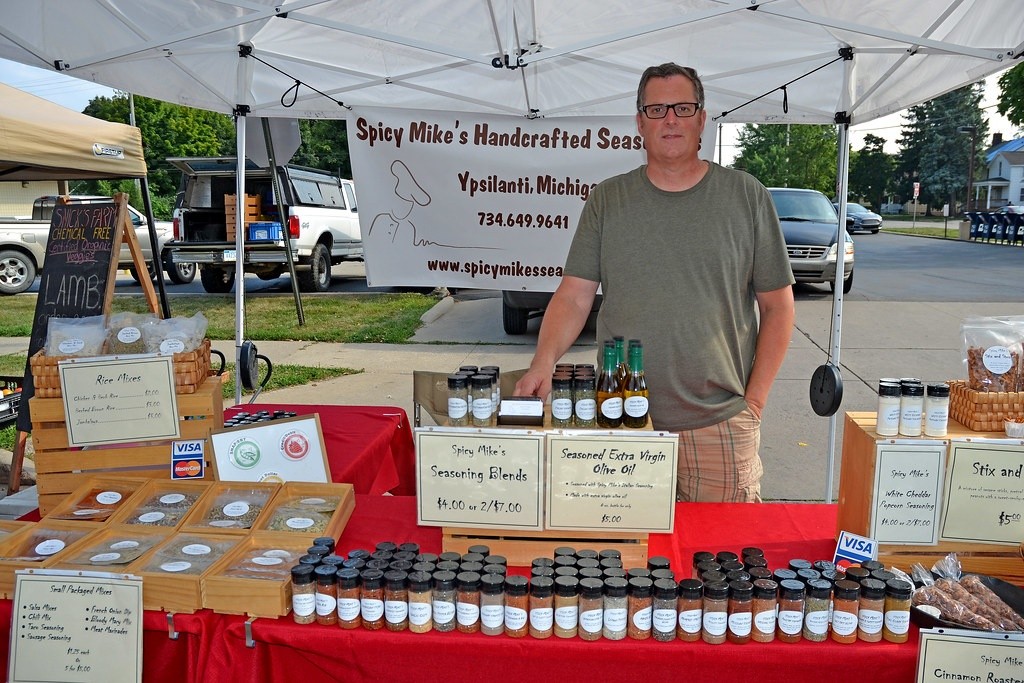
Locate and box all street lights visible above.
[956,125,977,213]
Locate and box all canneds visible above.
[875,378,951,438]
[551,363,597,428]
[447,365,501,427]
[291,536,913,644]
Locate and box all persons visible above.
[512,62,794,503]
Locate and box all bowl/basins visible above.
[908,569,1024,634]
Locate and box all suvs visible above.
[765,187,856,294]
[160,155,365,294]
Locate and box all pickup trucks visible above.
[0,194,197,297]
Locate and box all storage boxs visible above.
[833,410,1024,587]
[224,193,261,243]
[28,376,226,519]
[249,222,284,241]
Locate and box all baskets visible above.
[946,380,1024,432]
[31,336,211,397]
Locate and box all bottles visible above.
[291,537,913,644]
[875,377,925,437]
[595,335,649,429]
[223,409,296,430]
[551,362,596,428]
[447,365,501,427]
[924,383,950,438]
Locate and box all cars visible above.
[498,290,602,337]
[832,201,883,235]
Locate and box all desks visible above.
[1,403,1024,683]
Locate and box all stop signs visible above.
[914,183,920,196]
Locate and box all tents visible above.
[0,0,1024,502]
[0,81,172,321]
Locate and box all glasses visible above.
[640,102,701,119]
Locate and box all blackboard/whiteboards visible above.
[15,192,130,433]
